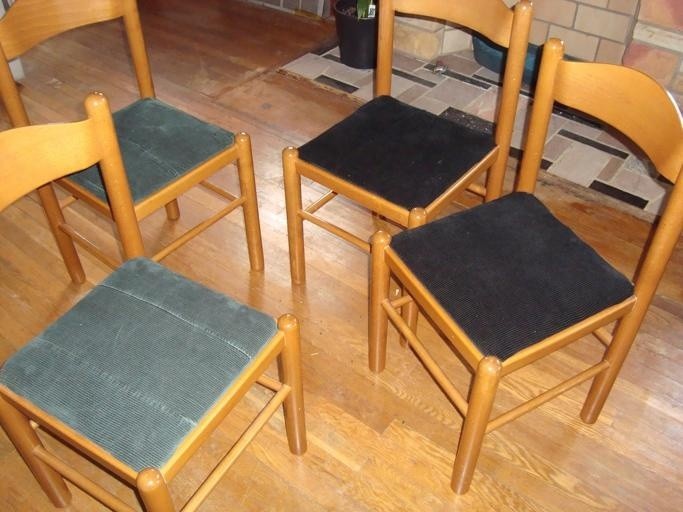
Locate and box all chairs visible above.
[281,0,534,350]
[368,37,683,492]
[0,92,307,511]
[1,0,265,285]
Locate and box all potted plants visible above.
[331,0,380,68]
